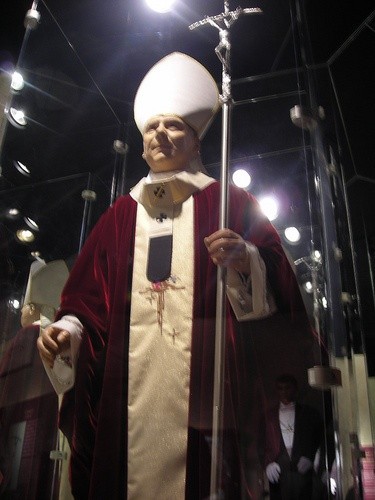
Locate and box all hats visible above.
[24,260,69,310]
[133,50,220,140]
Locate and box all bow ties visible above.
[281,401,296,409]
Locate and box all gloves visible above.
[297,456,312,474]
[266,462,280,481]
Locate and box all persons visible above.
[34,50,334,500]
[0,258,69,500]
[257,370,328,500]
